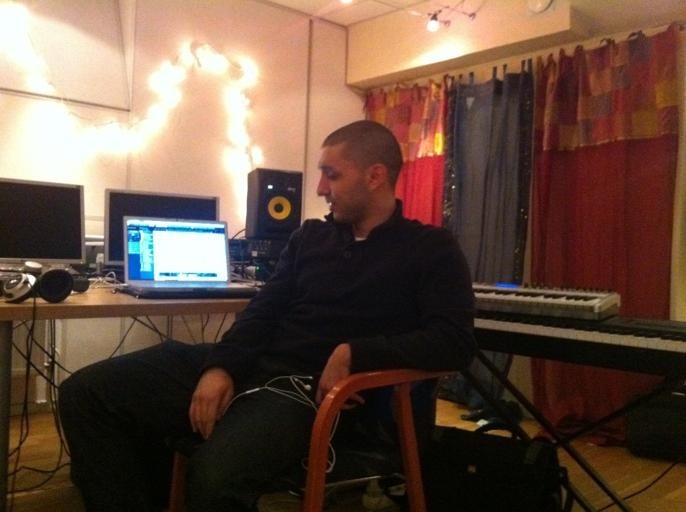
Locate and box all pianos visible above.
[472,280,621,321]
[474,317,686,374]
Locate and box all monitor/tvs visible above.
[0,178,86,265]
[104,188,220,267]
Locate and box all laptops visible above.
[122,216,259,299]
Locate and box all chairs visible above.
[169,369,454,511]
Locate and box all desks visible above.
[0,279,249,512]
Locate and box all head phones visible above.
[3,269,72,304]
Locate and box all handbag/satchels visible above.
[415,421,571,512]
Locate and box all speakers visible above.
[246,168,301,239]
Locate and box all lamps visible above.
[425,5,449,34]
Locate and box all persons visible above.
[58,120,475,510]
